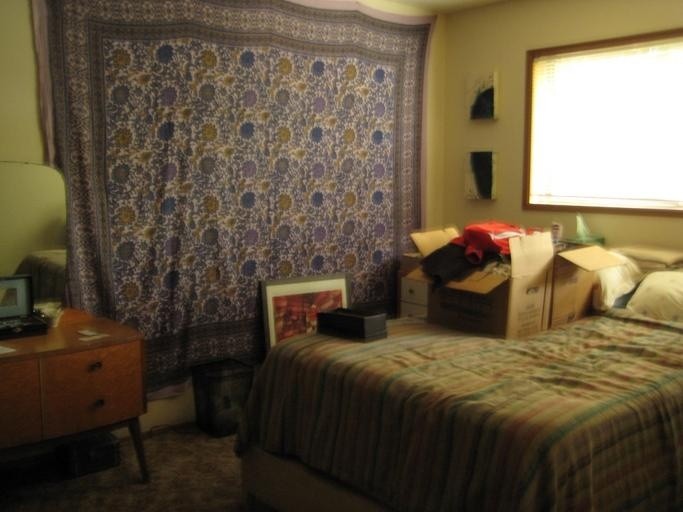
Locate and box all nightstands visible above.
[397,271,433,319]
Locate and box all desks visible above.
[0,308,150,483]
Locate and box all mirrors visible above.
[0,160,68,309]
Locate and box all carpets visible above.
[0,421,242,512]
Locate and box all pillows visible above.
[616,271,683,330]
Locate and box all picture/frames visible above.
[0,274,33,321]
[260,272,352,351]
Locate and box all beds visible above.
[234,247,683,512]
[13,249,67,303]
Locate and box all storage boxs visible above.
[410,227,629,338]
